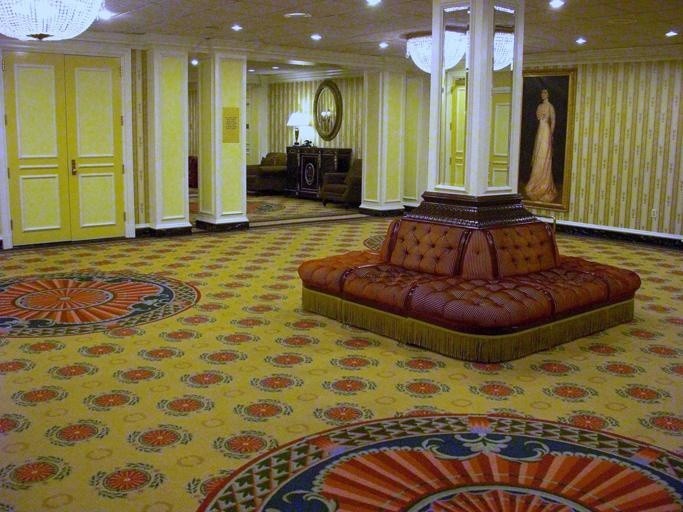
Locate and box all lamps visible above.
[0,0,102,41]
[402,27,466,75]
[286,112,308,147]
[466,26,514,72]
[320,110,330,122]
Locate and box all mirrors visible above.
[312,79,343,142]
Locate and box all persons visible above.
[523,85,561,204]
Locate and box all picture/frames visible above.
[522,65,578,213]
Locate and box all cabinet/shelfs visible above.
[283,147,351,201]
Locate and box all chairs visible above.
[246,152,288,197]
[318,159,362,210]
[188,156,198,188]
[298,216,642,363]
